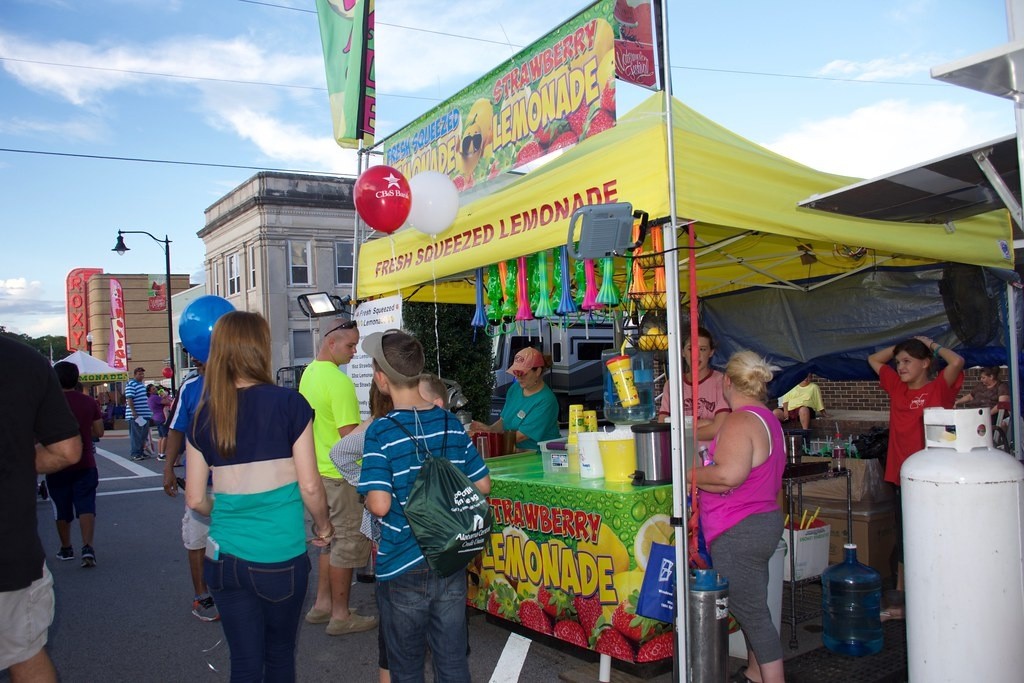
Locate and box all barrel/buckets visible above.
[628,420,673,485]
[599,440,637,482]
[578,432,608,479]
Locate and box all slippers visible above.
[729,665,760,683]
[880,607,905,622]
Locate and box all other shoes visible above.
[156,455,167,461]
[56,545,75,560]
[129,452,150,460]
[80,544,96,567]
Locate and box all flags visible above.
[316,0,375,149]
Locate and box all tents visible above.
[52,350,129,407]
[350,90,1024,683]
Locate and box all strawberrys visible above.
[727,613,740,633]
[454,77,616,191]
[468,582,674,662]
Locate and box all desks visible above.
[468,449,678,682]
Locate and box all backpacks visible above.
[386,412,494,579]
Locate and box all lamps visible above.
[297,292,351,318]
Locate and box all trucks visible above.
[488,314,649,429]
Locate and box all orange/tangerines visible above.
[502,514,676,622]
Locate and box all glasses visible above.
[325,319,357,336]
[147,384,152,391]
[137,368,145,372]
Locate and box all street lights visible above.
[86,332,95,398]
[43,338,53,366]
[109,229,176,400]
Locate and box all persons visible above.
[35,361,105,569]
[867,335,964,622]
[772,372,834,430]
[186,312,335,683]
[0,334,82,683]
[657,327,732,466]
[952,366,1010,442]
[330,329,491,683]
[125,347,220,622]
[467,347,562,453]
[297,319,380,636]
[685,352,786,683]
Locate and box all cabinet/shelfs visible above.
[782,469,853,654]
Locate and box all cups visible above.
[607,355,639,408]
[569,405,598,473]
[473,429,518,458]
[786,435,802,467]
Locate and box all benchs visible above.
[811,410,889,428]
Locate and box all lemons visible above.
[638,326,669,351]
[435,18,615,172]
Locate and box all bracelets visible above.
[930,342,936,353]
[305,521,335,545]
[933,343,942,359]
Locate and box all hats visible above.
[505,347,545,378]
[362,328,426,383]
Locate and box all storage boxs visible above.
[536,437,569,473]
[786,456,883,501]
[784,497,902,566]
[782,524,830,582]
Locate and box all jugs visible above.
[664,416,698,469]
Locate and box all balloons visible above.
[406,170,459,238]
[353,165,411,235]
[179,295,237,364]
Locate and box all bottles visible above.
[832,432,846,477]
[700,446,734,496]
[821,542,884,656]
[602,349,657,426]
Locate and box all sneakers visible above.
[191,594,220,621]
[305,605,333,624]
[325,608,376,635]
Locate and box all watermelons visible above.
[613,0,656,89]
